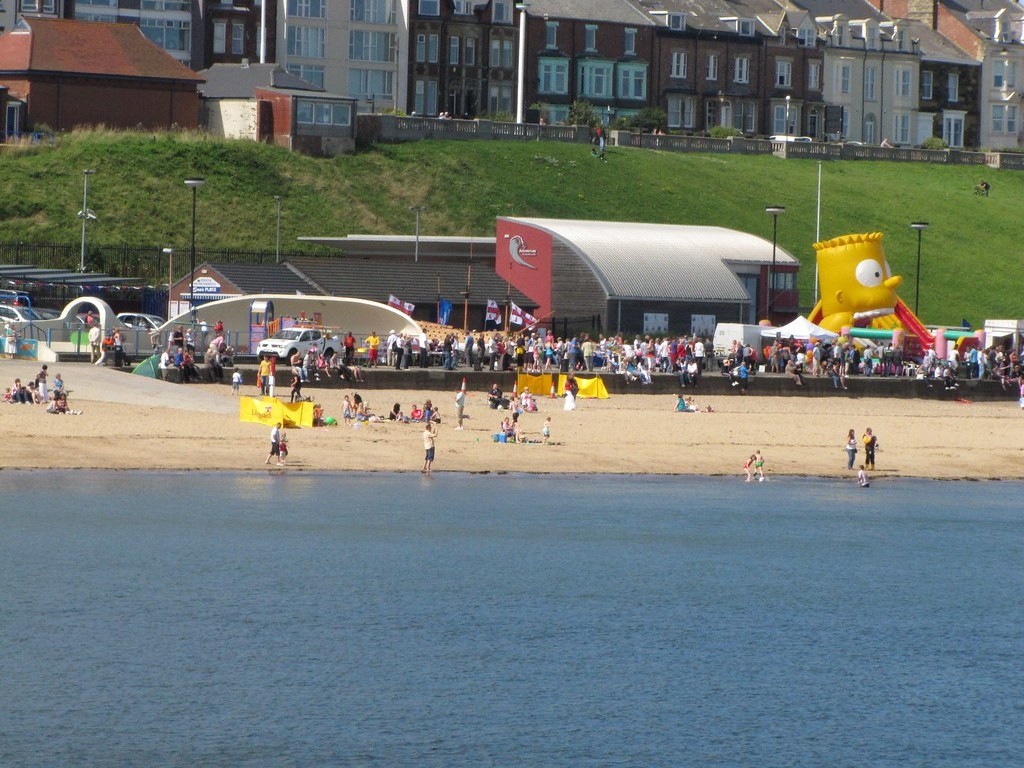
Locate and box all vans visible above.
[714,324,783,365]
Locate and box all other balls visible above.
[325,417,335,424]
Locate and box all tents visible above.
[760,316,839,344]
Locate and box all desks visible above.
[392,352,420,366]
[429,351,453,367]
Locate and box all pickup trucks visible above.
[256,329,342,368]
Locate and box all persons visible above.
[979,179,990,197]
[652,128,665,148]
[597,124,606,152]
[591,148,605,159]
[2,311,1024,486]
[437,112,452,120]
[880,138,892,147]
[421,424,439,474]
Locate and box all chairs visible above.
[352,347,368,366]
[903,361,917,377]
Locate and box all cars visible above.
[0,302,102,331]
[117,314,164,329]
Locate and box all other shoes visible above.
[277,463,284,466]
[266,461,270,465]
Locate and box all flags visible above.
[439,298,452,325]
[486,299,502,325]
[509,301,537,331]
[388,294,415,317]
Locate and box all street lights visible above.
[273,195,283,262]
[80,168,96,273]
[516,2,531,130]
[183,178,206,305]
[910,221,929,316]
[765,205,785,325]
[163,248,174,319]
[786,94,791,137]
[409,205,427,261]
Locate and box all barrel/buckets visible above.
[499,434,507,443]
[493,433,499,442]
